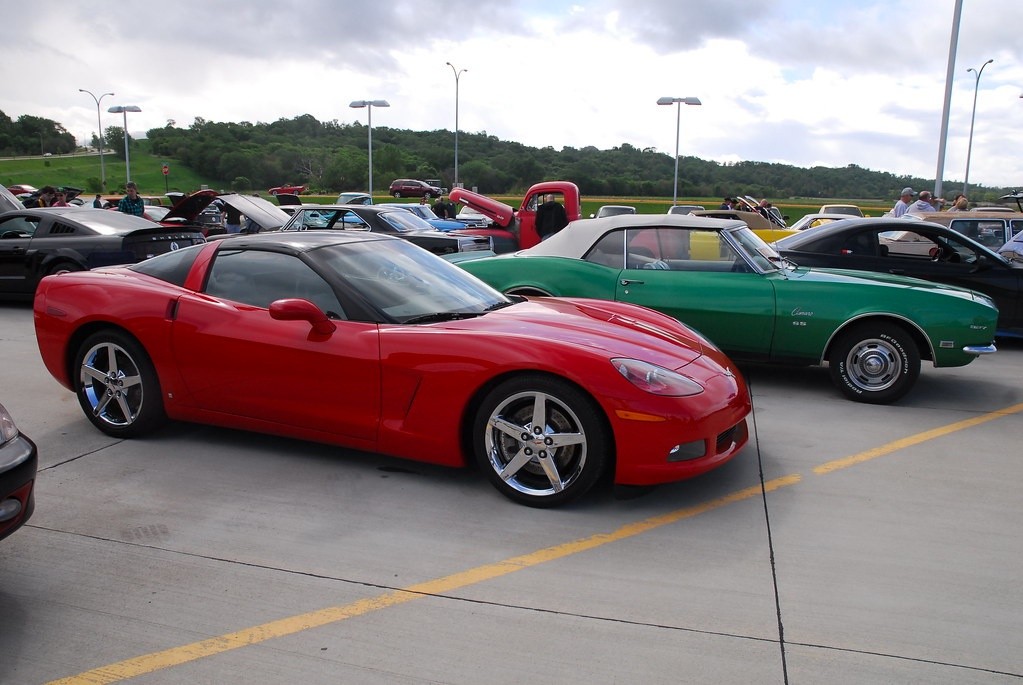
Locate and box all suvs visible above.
[388,179,443,200]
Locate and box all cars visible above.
[44,152,52,157]
[0,403,39,541]
[1,180,1023,259]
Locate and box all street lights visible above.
[80,88,116,191]
[349,100,390,196]
[657,96,702,205]
[964,58,994,195]
[108,105,142,184]
[446,61,467,186]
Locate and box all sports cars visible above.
[1,188,221,307]
[763,217,1023,352]
[433,214,1001,406]
[33,229,754,509]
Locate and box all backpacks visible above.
[882,208,895,218]
[22,198,45,209]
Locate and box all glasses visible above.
[46,194,53,197]
[911,195,914,197]
[930,197,931,199]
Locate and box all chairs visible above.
[878,244,889,257]
[1,231,21,240]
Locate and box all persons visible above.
[93,194,102,208]
[432,197,456,219]
[890,187,943,217]
[720,197,767,213]
[221,192,261,233]
[535,194,568,240]
[947,194,969,213]
[24,185,70,208]
[118,182,144,216]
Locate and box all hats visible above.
[731,199,738,203]
[56,187,68,193]
[96,194,102,198]
[901,187,918,197]
[724,196,731,201]
[127,181,136,190]
[931,193,938,199]
[435,199,441,203]
